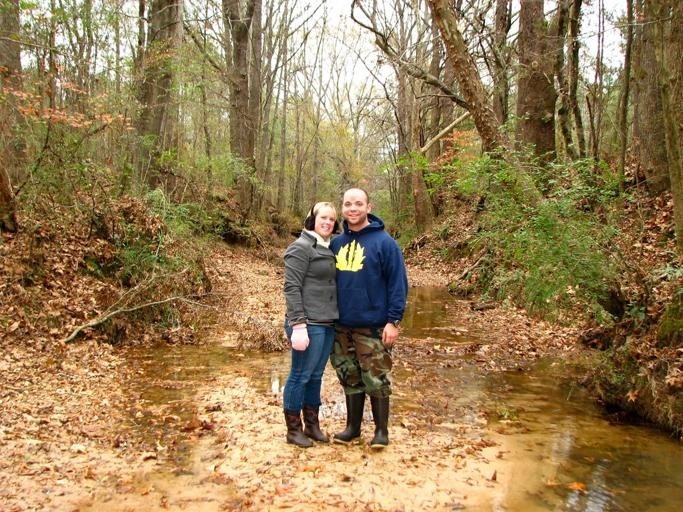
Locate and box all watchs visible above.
[388,319,400,327]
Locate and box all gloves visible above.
[291,324,310,352]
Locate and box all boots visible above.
[334,392,365,444]
[284,411,313,447]
[370,395,389,449]
[302,406,329,442]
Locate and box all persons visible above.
[281,200,343,448]
[328,186,410,450]
